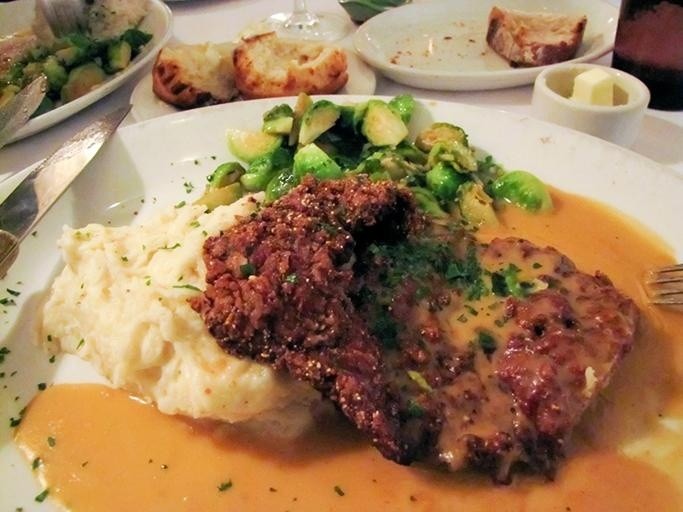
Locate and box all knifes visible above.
[0,74,49,149]
[0,104,132,280]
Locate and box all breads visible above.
[485,6,587,66]
[231,31,348,97]
[150,39,241,108]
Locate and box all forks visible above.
[645,264,683,304]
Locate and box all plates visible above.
[0,93,683,512]
[352,0,619,91]
[0,0,174,146]
[533,60,651,151]
[127,0,376,123]
